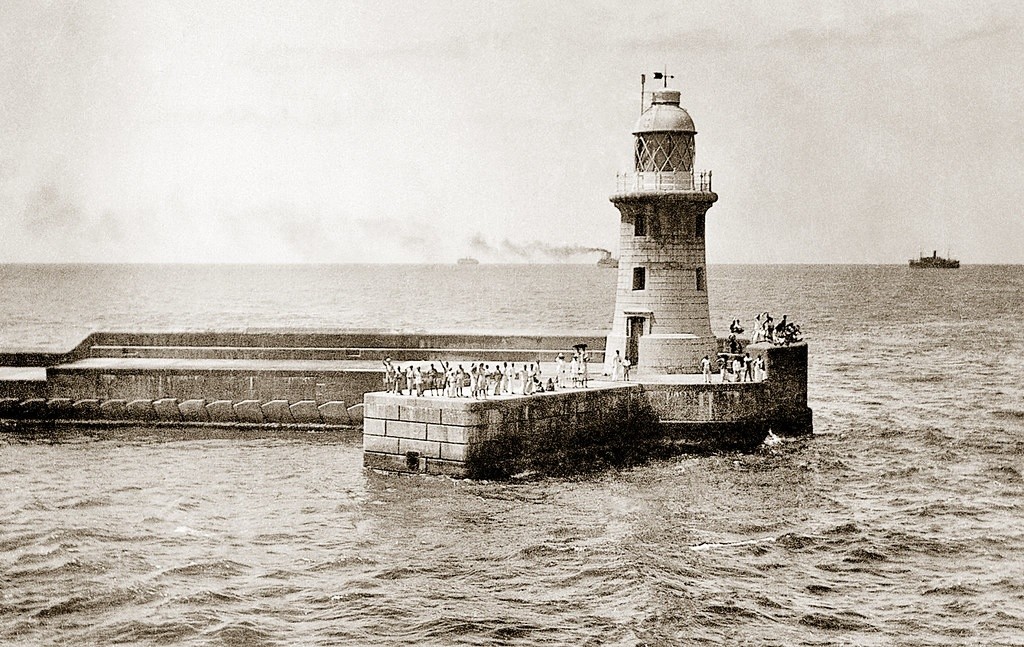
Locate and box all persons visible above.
[700,354,712,383]
[716,353,768,382]
[518,359,545,395]
[611,350,623,381]
[622,355,633,381]
[381,355,465,398]
[470,361,517,399]
[753,311,803,346]
[730,319,745,334]
[546,344,591,391]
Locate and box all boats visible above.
[458,257,479,266]
[908,250,962,270]
[596,250,619,267]
